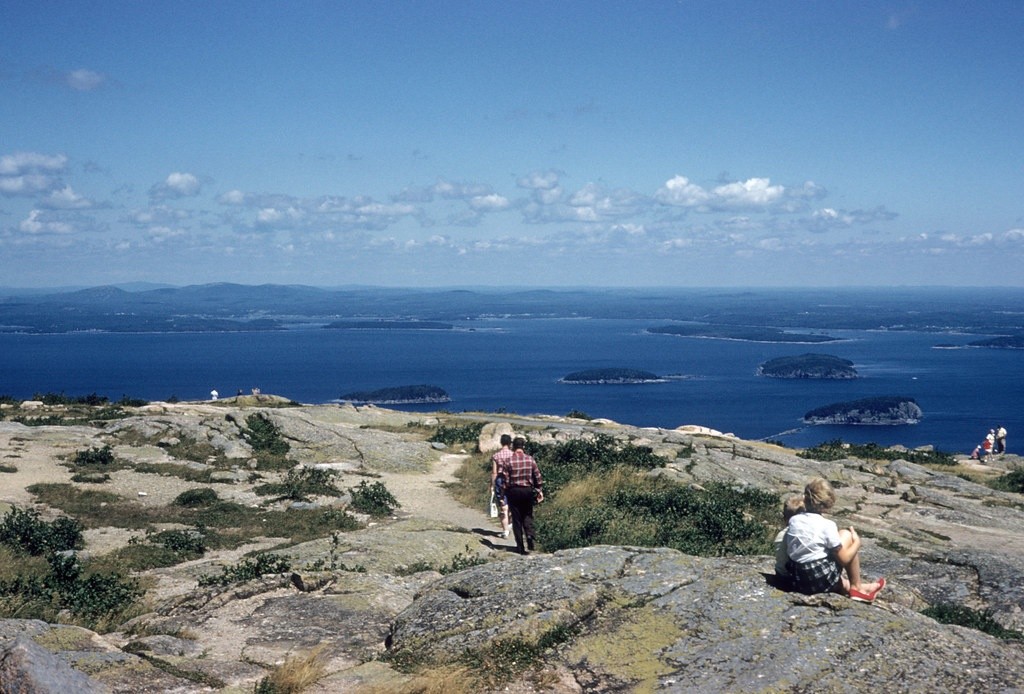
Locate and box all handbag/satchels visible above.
[490,492,498,518]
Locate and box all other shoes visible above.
[502,530,510,539]
[516,548,525,554]
[849,589,874,603]
[527,535,535,550]
[870,578,886,599]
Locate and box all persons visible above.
[499,437,544,554]
[971,424,1007,462]
[774,480,886,602]
[490,434,513,539]
[211,388,219,401]
[237,389,242,396]
[252,387,260,395]
